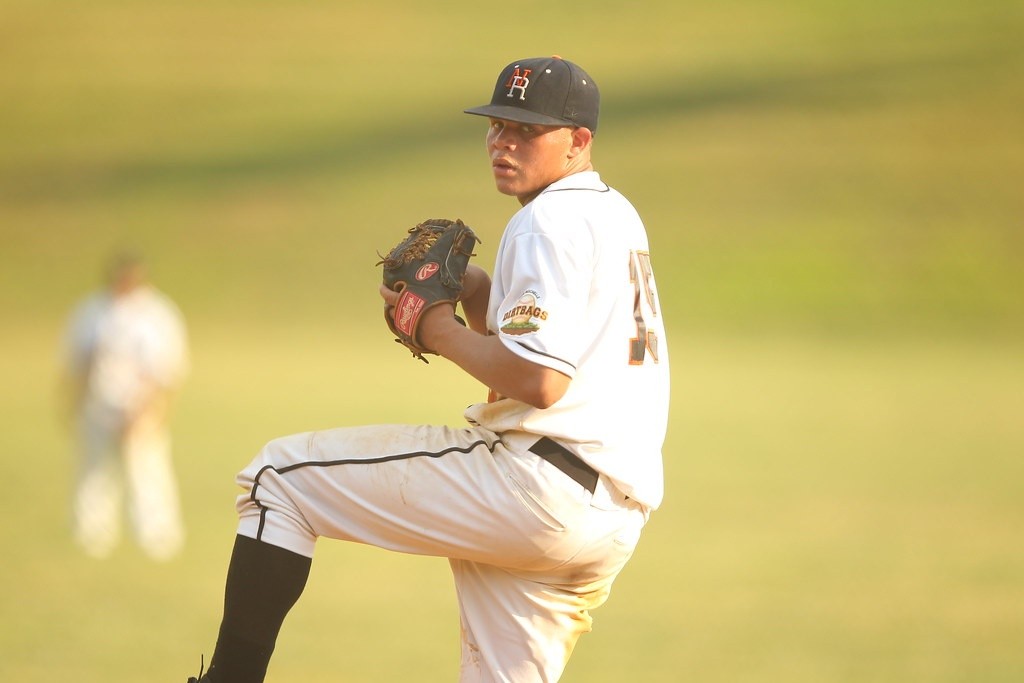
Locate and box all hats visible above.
[463,55,598,137]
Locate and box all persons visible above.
[64,249,195,566]
[185,56,670,682]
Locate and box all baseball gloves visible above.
[376,219,483,364]
